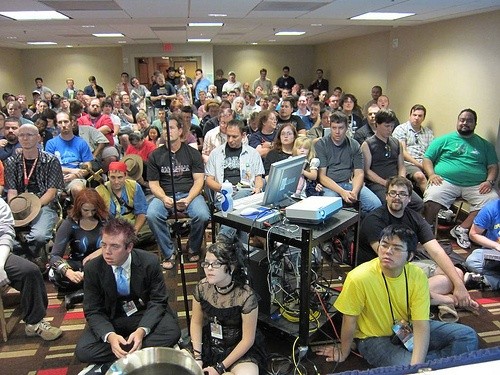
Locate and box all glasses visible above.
[201,262,229,268]
[387,190,410,198]
[280,132,292,136]
[19,133,37,137]
[268,117,278,121]
[414,134,419,144]
[385,145,389,157]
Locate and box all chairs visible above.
[0,187,500,343]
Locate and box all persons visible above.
[392,104,457,223]
[180,243,267,375]
[464,181,500,291]
[50,188,110,310]
[75,218,181,375]
[0,198,62,340]
[357,176,479,310]
[423,108,499,248]
[316,223,478,367]
[0,66,423,274]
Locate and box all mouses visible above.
[241,208,259,216]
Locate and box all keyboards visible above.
[233,192,265,210]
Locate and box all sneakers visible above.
[25,319,62,340]
[450,224,471,248]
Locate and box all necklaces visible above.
[214,281,233,290]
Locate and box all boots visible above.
[65,290,84,311]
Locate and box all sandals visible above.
[162,250,178,270]
[187,243,200,262]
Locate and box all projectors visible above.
[286,196,342,224]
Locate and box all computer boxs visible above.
[242,243,286,314]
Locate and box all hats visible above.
[166,67,178,72]
[8,193,42,227]
[109,162,127,172]
[228,71,236,76]
[121,154,143,181]
[33,90,40,94]
[0,111,7,119]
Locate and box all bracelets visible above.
[488,180,495,185]
[193,349,202,361]
[191,340,203,344]
[214,362,226,375]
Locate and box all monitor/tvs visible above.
[262,154,307,208]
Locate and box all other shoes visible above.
[438,209,453,219]
[174,344,193,356]
[78,364,102,375]
[49,269,55,281]
[463,272,483,290]
[438,305,459,323]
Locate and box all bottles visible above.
[159,94,166,105]
[221,179,234,217]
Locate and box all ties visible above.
[115,267,130,296]
